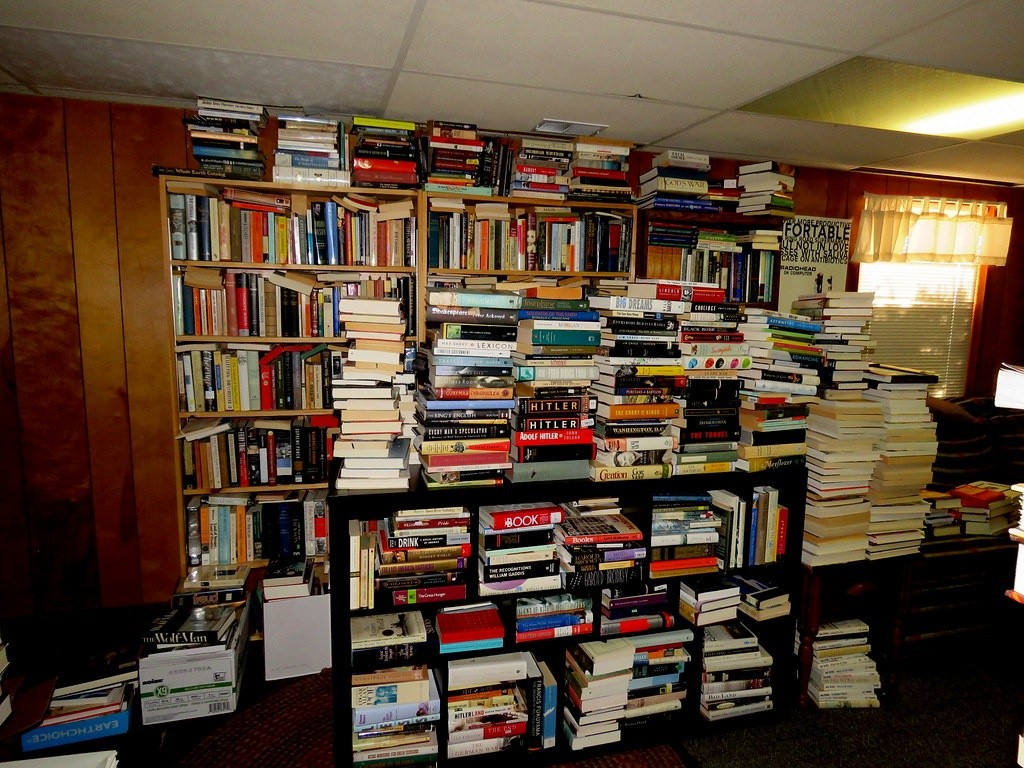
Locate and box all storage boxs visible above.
[138,588,252,726]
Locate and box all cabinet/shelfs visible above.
[637,208,784,311]
[797,533,1021,707]
[423,190,637,349]
[324,467,811,768]
[160,173,421,578]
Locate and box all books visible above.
[2,91,1024,767]
